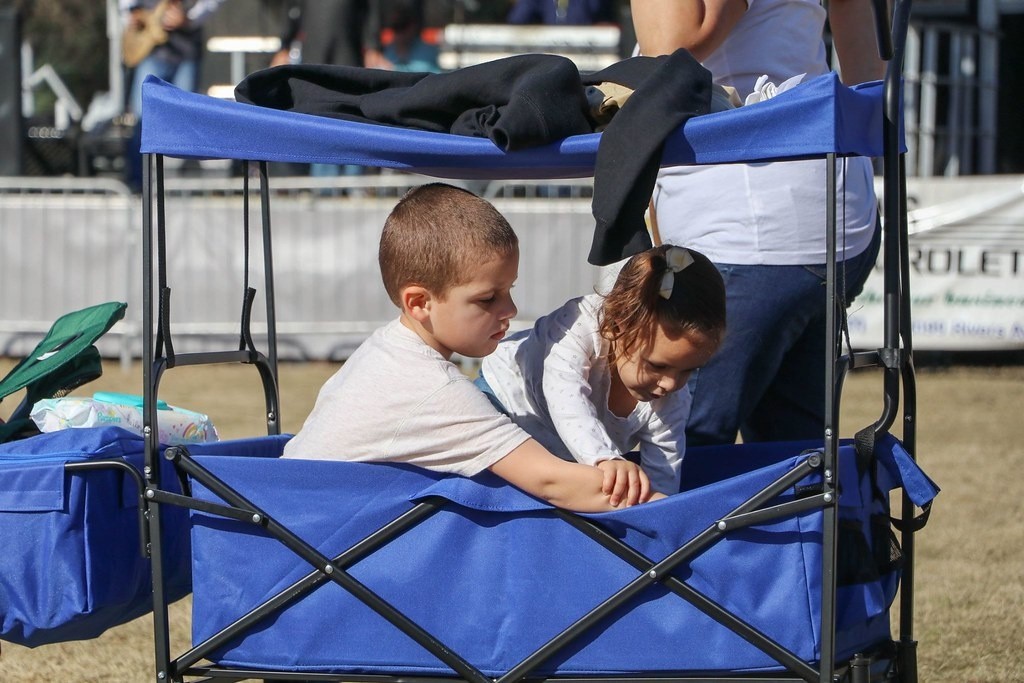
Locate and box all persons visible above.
[471,240,729,510]
[599,1,890,446]
[271,181,672,514]
[116,0,599,200]
